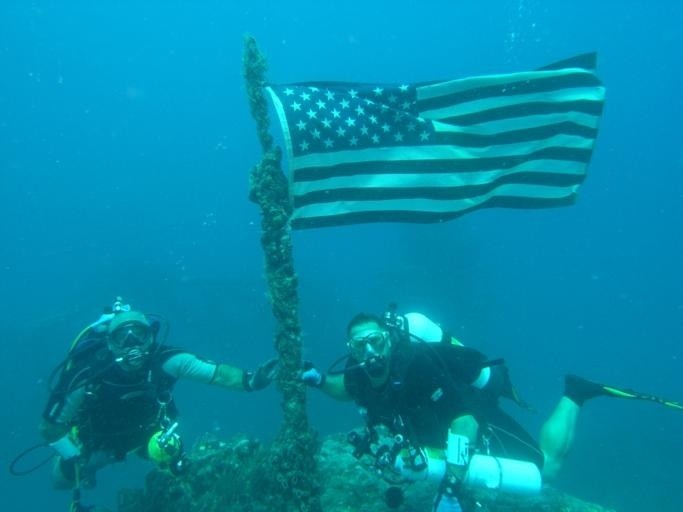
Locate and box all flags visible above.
[260,50,608,231]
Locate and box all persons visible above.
[300,312,610,494]
[38,310,279,491]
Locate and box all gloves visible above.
[432,491,463,512]
[292,361,325,388]
[240,356,282,392]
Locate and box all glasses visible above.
[102,323,155,347]
[346,331,391,356]
